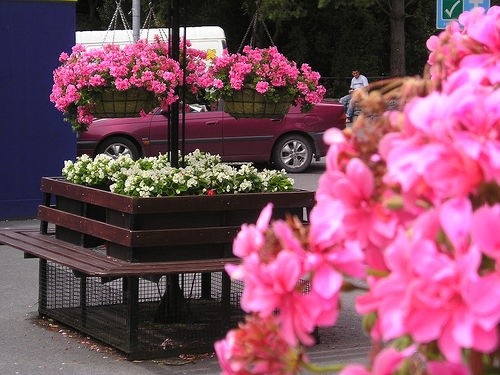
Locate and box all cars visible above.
[76,97,347,174]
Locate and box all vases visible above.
[221,87,293,122]
[87,84,157,120]
[35,175,317,258]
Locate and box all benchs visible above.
[101,257,256,284]
[0,225,132,282]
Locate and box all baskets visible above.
[88,87,151,117]
[225,85,289,116]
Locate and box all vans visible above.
[74,24,228,72]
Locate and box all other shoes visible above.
[345,117,353,123]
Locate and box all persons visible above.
[339,68,369,122]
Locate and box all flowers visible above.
[214,4,500,375]
[205,44,328,114]
[59,147,297,198]
[49,34,205,137]
